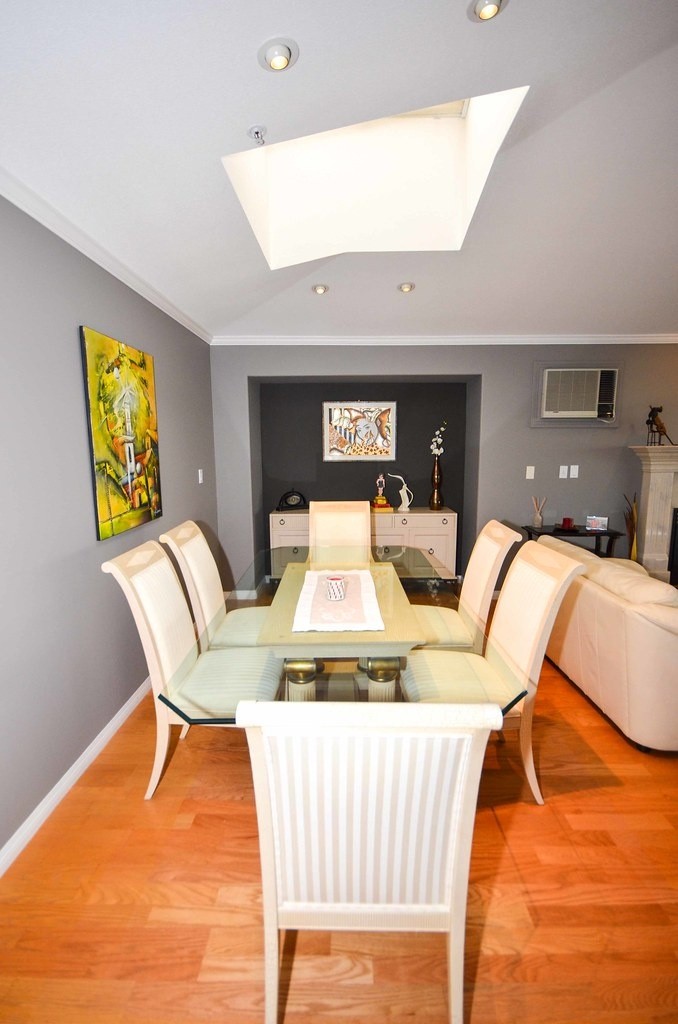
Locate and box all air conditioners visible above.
[541,368,618,423]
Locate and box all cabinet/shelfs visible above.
[269,508,457,595]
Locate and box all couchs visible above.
[537,534,678,753]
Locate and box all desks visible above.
[521,524,626,557]
[158,545,528,712]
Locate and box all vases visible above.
[429,453,444,510]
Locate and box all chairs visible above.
[305,500,376,563]
[235,700,503,1023]
[101,540,285,800]
[411,518,523,655]
[398,539,586,804]
[159,519,271,653]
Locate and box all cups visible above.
[326,575,345,600]
[563,518,571,528]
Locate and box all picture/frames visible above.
[79,325,162,540]
[322,400,396,462]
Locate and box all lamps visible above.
[402,283,411,292]
[316,285,325,294]
[265,43,291,70]
[474,0,501,19]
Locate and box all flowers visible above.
[430,420,447,456]
